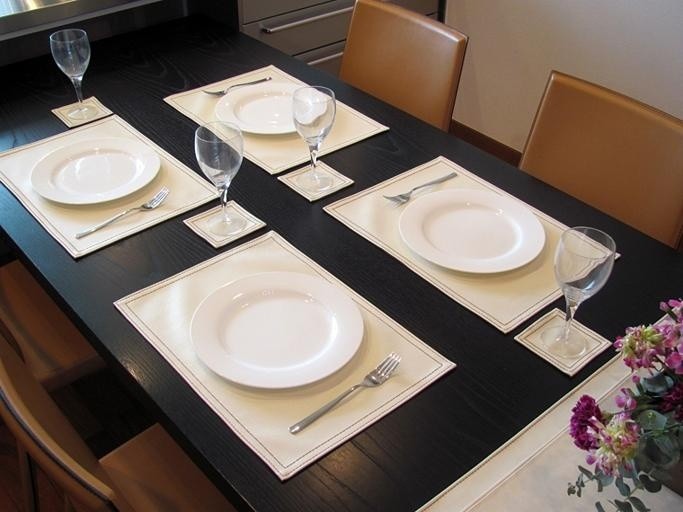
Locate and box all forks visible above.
[286,351,403,434]
[382,170,459,205]
[73,185,171,240]
[201,76,272,97]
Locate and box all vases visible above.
[627,441,680,499]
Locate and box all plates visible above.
[27,136,162,207]
[396,187,549,274]
[213,80,329,137]
[188,270,366,391]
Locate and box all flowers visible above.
[566,297,681,512]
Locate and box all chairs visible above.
[517,69,681,251]
[0,257,110,510]
[0,325,239,512]
[340,0,468,131]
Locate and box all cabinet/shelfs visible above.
[190,0,446,91]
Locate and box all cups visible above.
[194,120,244,239]
[47,26,100,122]
[291,83,337,193]
[539,225,616,360]
